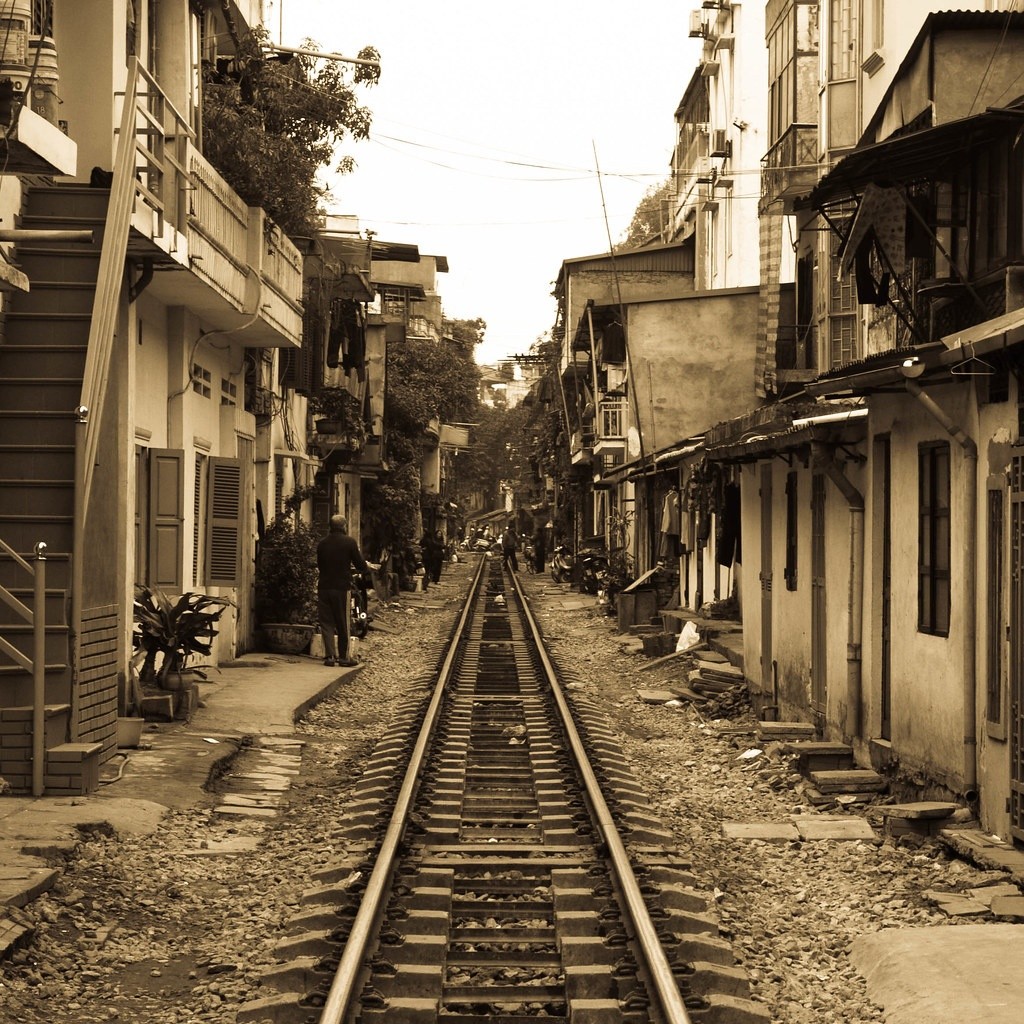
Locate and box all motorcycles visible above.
[548,543,576,584]
[350,563,381,638]
[577,546,609,595]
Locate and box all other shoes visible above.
[341,659,358,667]
[324,661,335,667]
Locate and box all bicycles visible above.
[522,547,538,576]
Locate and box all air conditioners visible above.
[690,0,716,37]
[708,129,727,157]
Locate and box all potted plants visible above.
[254,483,329,654]
[396,547,425,591]
[310,388,348,435]
[132,582,241,691]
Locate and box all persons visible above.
[420,529,446,593]
[502,524,521,571]
[457,526,490,550]
[530,528,545,574]
[316,515,382,667]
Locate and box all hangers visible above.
[950,342,997,375]
[872,166,891,187]
[608,318,622,326]
[337,290,368,312]
